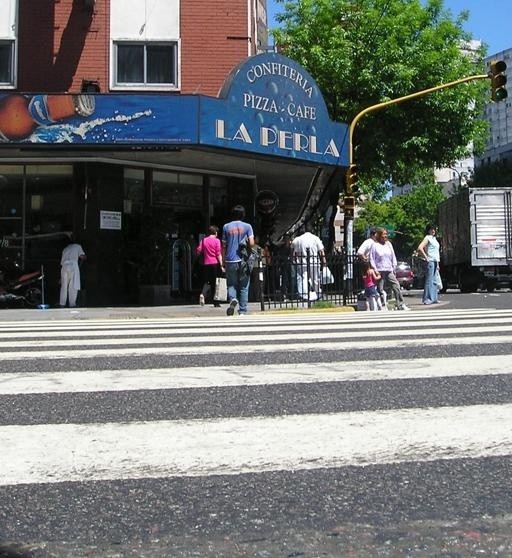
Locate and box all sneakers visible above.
[226,299,248,317]
[372,303,411,312]
[199,295,221,307]
[422,298,440,305]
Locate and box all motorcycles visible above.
[0,267,46,308]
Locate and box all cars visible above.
[395,261,415,290]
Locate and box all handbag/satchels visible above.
[212,276,229,302]
[433,263,444,291]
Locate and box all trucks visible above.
[437,184,511,294]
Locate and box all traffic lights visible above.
[490,60,508,102]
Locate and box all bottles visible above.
[0,94,96,142]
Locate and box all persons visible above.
[256,220,331,302]
[194,224,226,309]
[55,240,88,308]
[368,228,411,311]
[221,203,256,316]
[356,226,386,307]
[416,222,441,305]
[360,259,382,311]
[240,218,336,304]
